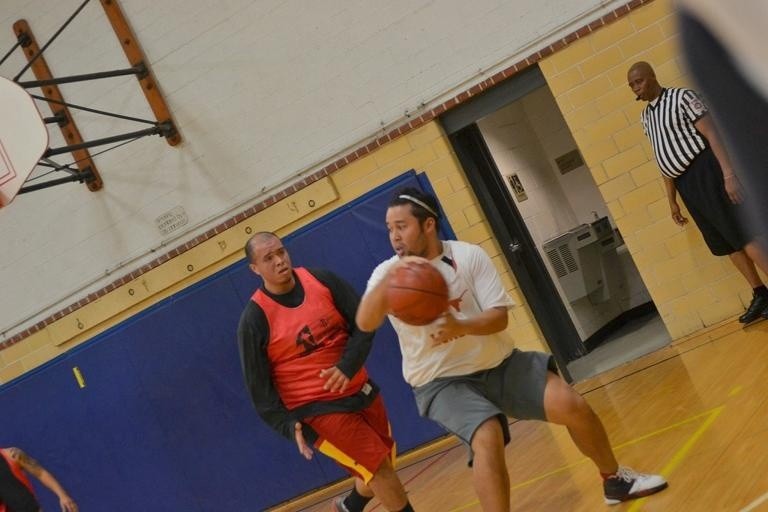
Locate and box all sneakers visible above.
[330,496,351,512]
[739,287,768,323]
[601,465,667,506]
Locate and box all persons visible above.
[238,230,416,511]
[0,446,79,512]
[626,61,768,328]
[353,184,671,511]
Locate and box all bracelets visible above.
[724,174,736,180]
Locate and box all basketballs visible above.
[386,262,448,325]
